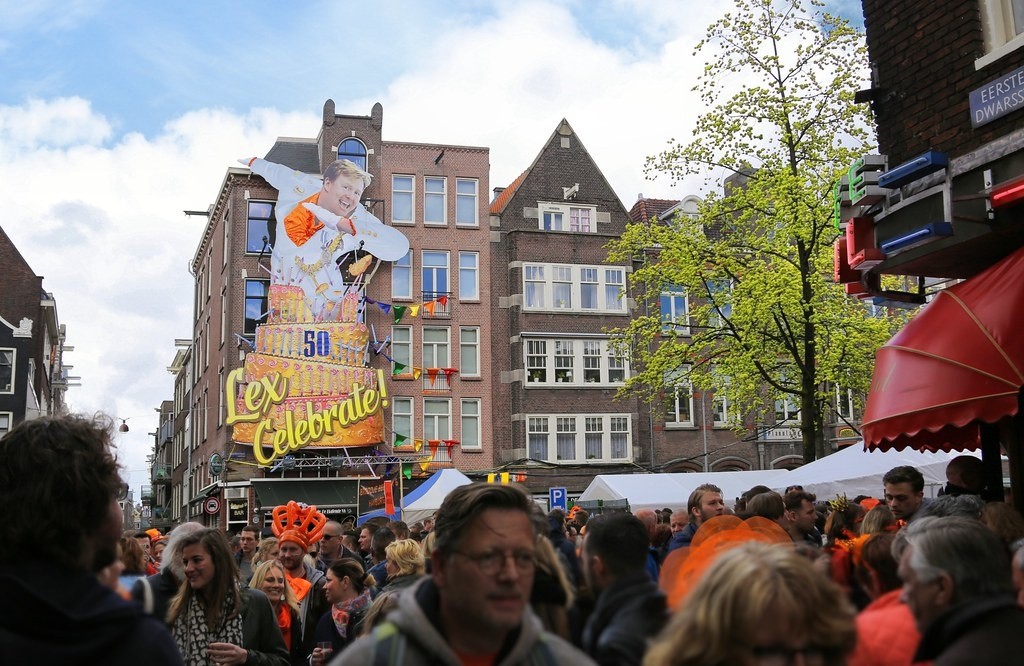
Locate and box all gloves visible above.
[302,202,343,232]
[235,156,258,167]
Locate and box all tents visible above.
[579,468,790,516]
[767,435,983,500]
[358,470,474,527]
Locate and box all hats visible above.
[270,499,327,554]
[144,528,163,542]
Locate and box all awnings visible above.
[859,246,1024,455]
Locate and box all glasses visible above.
[446,545,539,578]
[108,483,129,501]
[726,631,831,666]
[321,534,338,541]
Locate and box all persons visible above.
[166,527,289,666]
[892,515,1024,666]
[326,481,597,666]
[639,539,858,666]
[239,156,409,309]
[0,454,1024,666]
[0,415,184,666]
[130,521,205,622]
